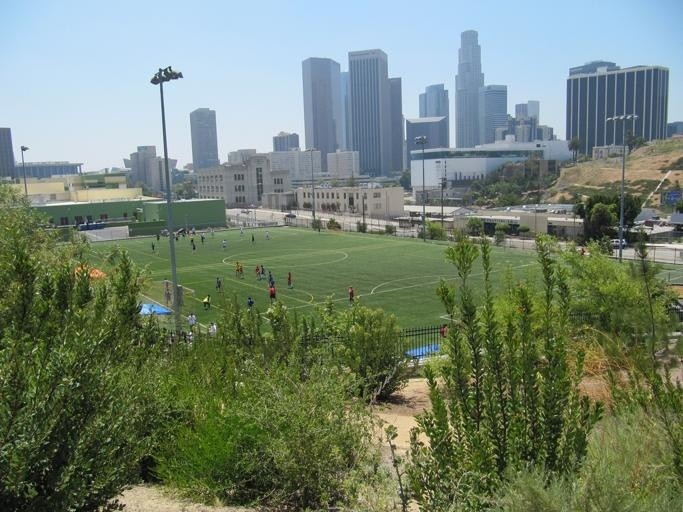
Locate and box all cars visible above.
[240,204,257,213]
[285,214,296,218]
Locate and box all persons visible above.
[348,286,356,305]
[443,323,449,340]
[287,270,293,290]
[163,278,171,290]
[150,220,273,251]
[440,326,444,338]
[183,260,276,345]
[165,289,173,306]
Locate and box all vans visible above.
[609,239,626,249]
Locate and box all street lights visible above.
[606,115,639,262]
[413,135,429,241]
[149,66,182,341]
[20,146,28,195]
[304,144,317,210]
